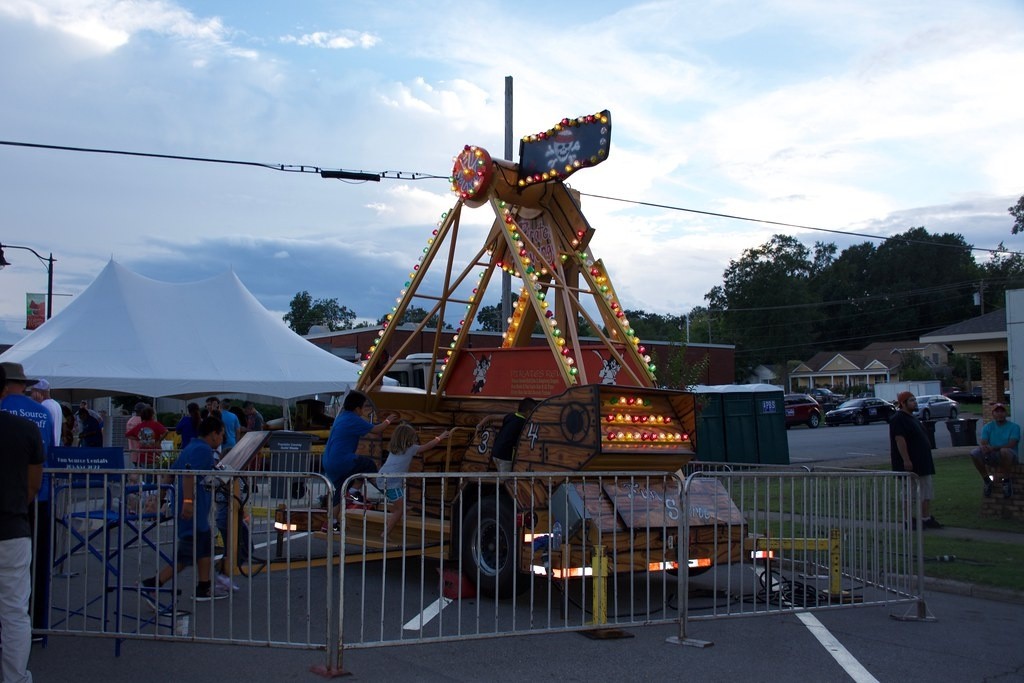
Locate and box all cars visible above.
[813,387,847,403]
[783,393,822,429]
[913,395,960,422]
[825,397,896,426]
[942,386,982,403]
[857,392,875,398]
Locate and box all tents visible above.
[0,262,399,463]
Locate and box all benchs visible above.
[360,410,505,504]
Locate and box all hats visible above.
[897,392,913,404]
[76,408,88,415]
[0,362,40,387]
[991,403,1006,413]
[98,409,107,414]
[134,402,150,412]
[33,379,50,390]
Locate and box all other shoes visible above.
[216,576,240,592]
[243,485,258,493]
[924,516,944,528]
[983,480,991,496]
[905,518,926,530]
[1002,479,1013,498]
[31,634,43,641]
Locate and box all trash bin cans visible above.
[923,420,938,449]
[945,419,979,446]
[271,430,317,498]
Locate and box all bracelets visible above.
[385,420,390,424]
[183,499,193,503]
[435,436,441,441]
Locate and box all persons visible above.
[890,390,944,531]
[972,403,1021,498]
[0,354,450,683]
[492,396,536,481]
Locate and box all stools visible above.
[49,444,177,655]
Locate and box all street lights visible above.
[0,243,57,321]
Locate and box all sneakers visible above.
[138,579,162,612]
[321,520,340,535]
[191,585,229,601]
[346,491,371,505]
